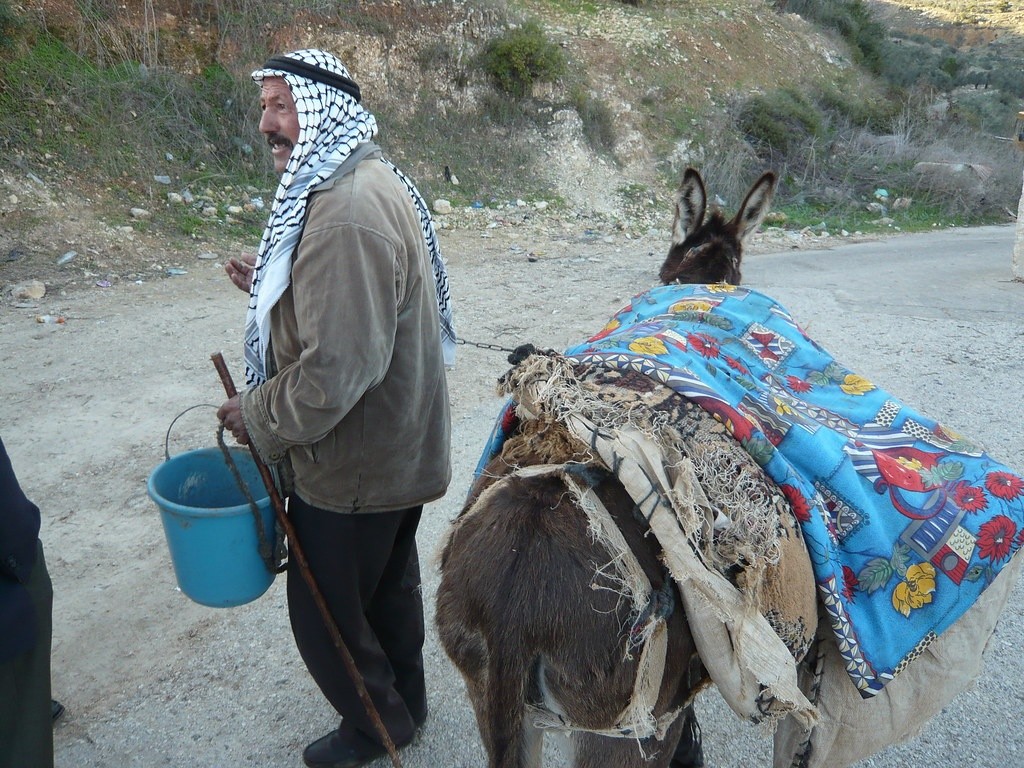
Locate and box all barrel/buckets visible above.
[148,403,283,609]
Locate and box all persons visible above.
[218,48,453,768]
[0,437,64,768]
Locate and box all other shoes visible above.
[303,727,422,768]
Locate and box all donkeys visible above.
[431,167,778,768]
